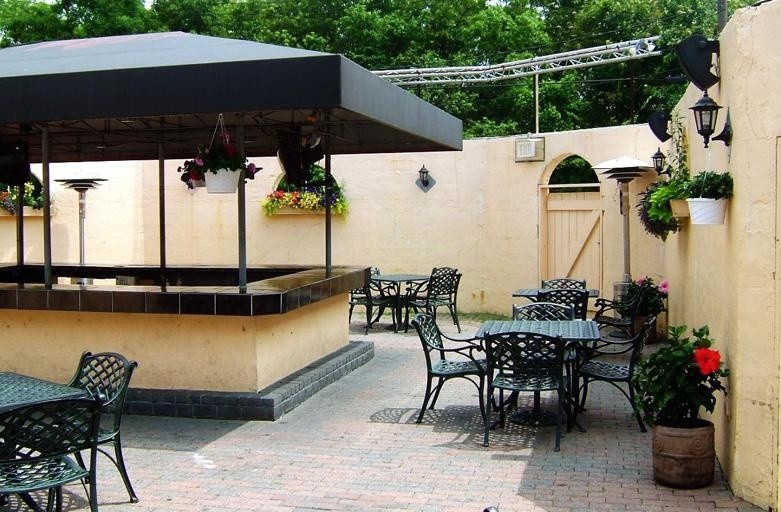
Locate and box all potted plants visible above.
[677,172,732,226]
[650,178,690,217]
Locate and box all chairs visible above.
[369,266,384,322]
[429,266,459,325]
[411,313,500,430]
[542,279,587,289]
[405,266,462,334]
[348,286,396,329]
[537,289,589,321]
[65,351,139,502]
[592,282,646,339]
[428,271,462,325]
[483,329,564,452]
[513,302,575,321]
[1,393,105,512]
[571,314,655,433]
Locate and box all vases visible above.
[653,419,714,490]
[628,314,656,345]
[204,167,238,193]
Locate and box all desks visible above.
[512,289,599,320]
[0,367,92,511]
[475,319,599,424]
[371,274,432,332]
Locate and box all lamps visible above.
[415,164,436,194]
[688,89,733,148]
[651,146,671,178]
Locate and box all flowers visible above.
[176,134,263,190]
[627,323,731,429]
[592,275,670,337]
